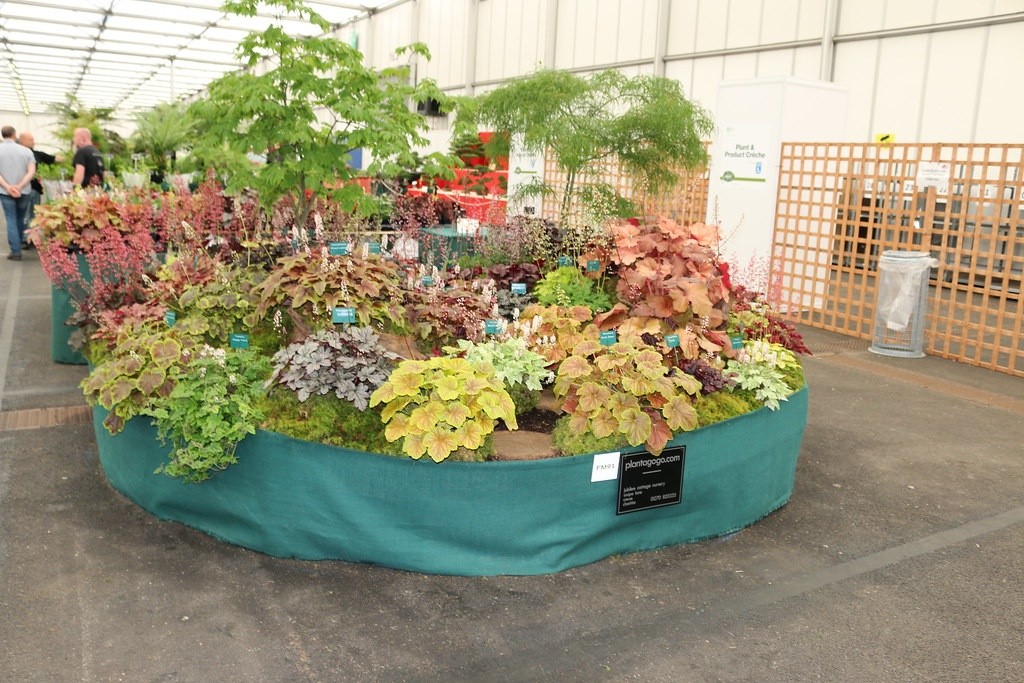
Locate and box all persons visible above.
[0,126,36,260]
[71,128,105,253]
[17,131,65,248]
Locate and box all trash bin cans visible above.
[869,250,933,358]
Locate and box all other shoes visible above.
[19,241,28,249]
[7,254,21,260]
